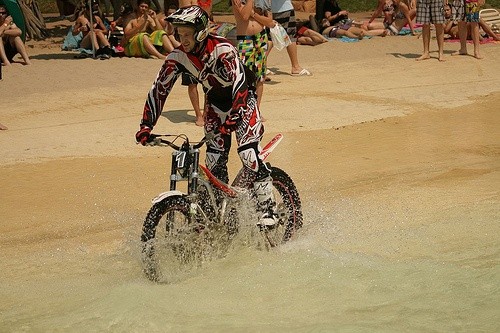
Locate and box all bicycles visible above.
[134,133,303,285]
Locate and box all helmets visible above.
[163,5,209,43]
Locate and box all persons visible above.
[296,0,500,46]
[135,5,282,228]
[408,0,449,62]
[179,0,213,127]
[65,0,181,60]
[232,0,276,123]
[0,6,31,67]
[451,0,485,59]
[264,0,313,76]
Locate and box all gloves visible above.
[135,123,151,146]
[219,112,243,135]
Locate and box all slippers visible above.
[291,69,312,76]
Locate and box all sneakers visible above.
[256,205,283,229]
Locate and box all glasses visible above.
[0,12,6,15]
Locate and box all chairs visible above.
[478,9,500,36]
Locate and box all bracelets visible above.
[4,23,8,26]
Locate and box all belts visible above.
[237,29,265,40]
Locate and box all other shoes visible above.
[102,46,112,59]
[96,49,103,59]
